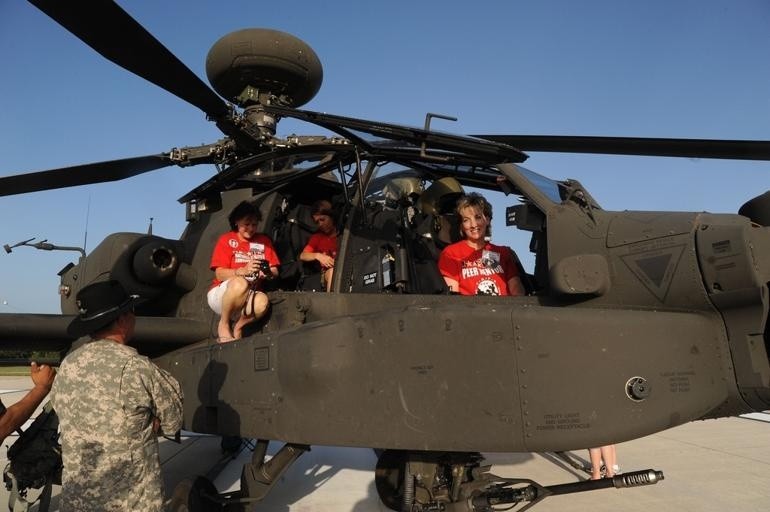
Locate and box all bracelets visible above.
[232,268,239,276]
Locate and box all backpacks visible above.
[5,401,63,512]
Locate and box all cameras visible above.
[260,259,271,275]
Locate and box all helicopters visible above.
[0,0,770,512]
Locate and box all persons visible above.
[439,190,526,296]
[0,358,56,443]
[49,282,185,512]
[300,201,341,292]
[205,202,280,341]
[586,444,624,476]
[585,443,614,480]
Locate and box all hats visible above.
[67,280,149,340]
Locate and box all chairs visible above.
[290,206,321,292]
